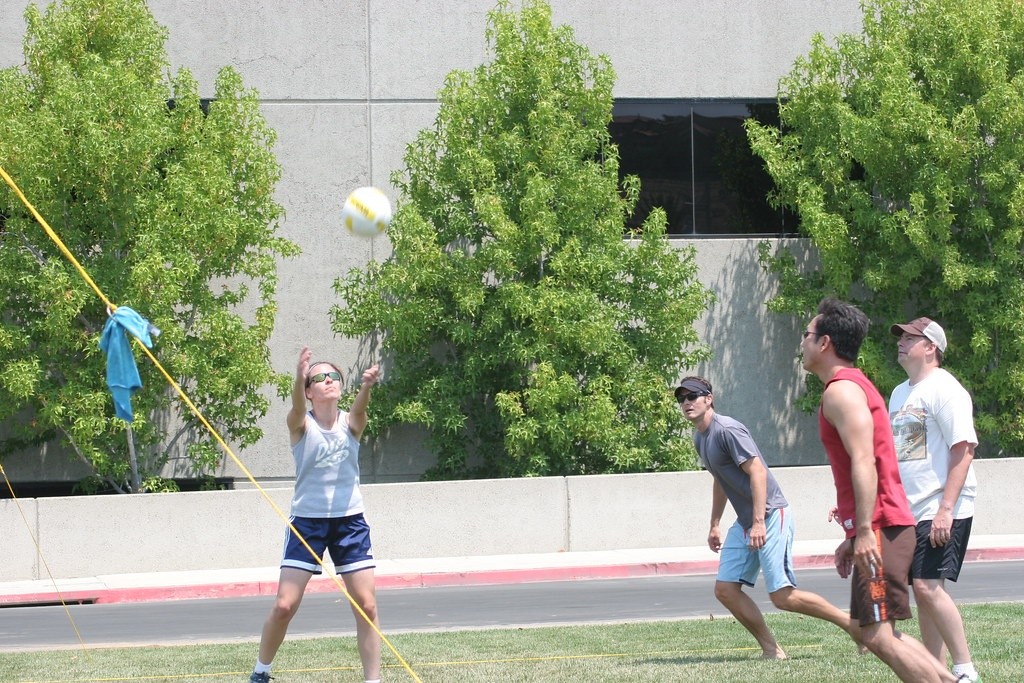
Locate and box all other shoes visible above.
[249,672,274,683]
[955,674,983,683]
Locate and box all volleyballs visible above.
[342,184,393,239]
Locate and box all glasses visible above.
[306,372,340,387]
[803,331,824,338]
[678,392,708,403]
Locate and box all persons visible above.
[801,295,973,683]
[674,377,868,661]
[829,316,978,683]
[250,345,381,683]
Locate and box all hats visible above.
[891,317,947,352]
[675,379,711,396]
